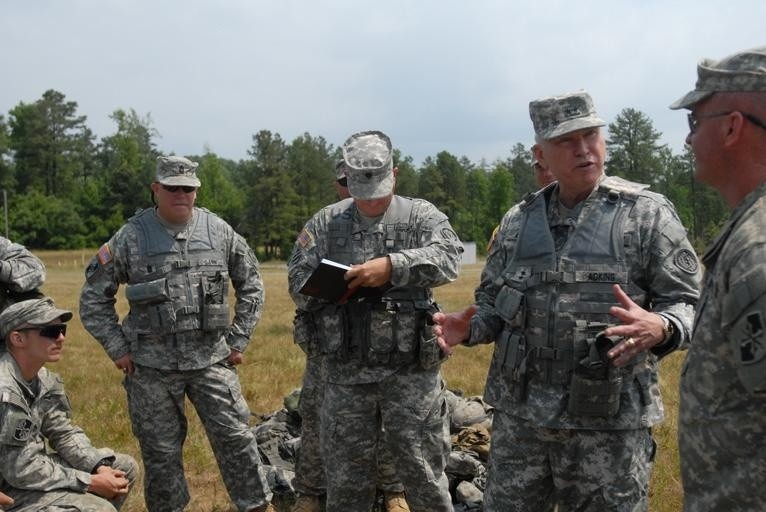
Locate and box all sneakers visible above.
[383,491,410,512]
[291,494,321,511]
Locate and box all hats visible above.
[336,159,346,181]
[669,46,766,109]
[155,156,201,187]
[0,297,72,340]
[529,90,605,142]
[343,130,394,200]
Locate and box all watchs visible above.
[93,457,113,474]
[651,314,675,348]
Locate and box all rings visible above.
[627,338,635,348]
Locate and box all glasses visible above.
[687,110,766,134]
[16,323,67,339]
[336,177,347,187]
[163,185,194,193]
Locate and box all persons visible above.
[287,129,456,511]
[80,156,274,512]
[670,44,766,512]
[530,144,556,187]
[291,158,411,512]
[1,236,46,313]
[0,491,14,506]
[0,295,140,511]
[431,90,710,512]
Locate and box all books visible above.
[297,258,393,305]
